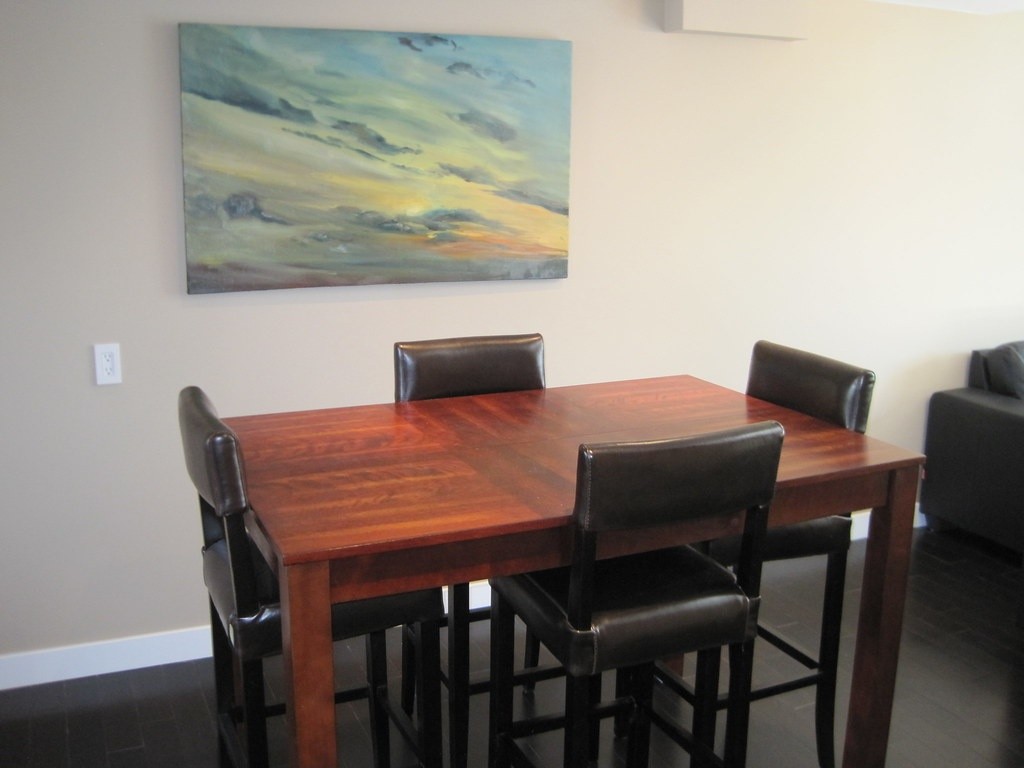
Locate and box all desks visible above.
[220,373,926,768]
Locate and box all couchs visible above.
[922,348,1024,554]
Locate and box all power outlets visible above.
[94,341,123,385]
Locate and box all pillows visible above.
[987,341,1024,400]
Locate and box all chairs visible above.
[624,339,877,768]
[177,386,445,768]
[487,418,782,768]
[395,334,625,739]
